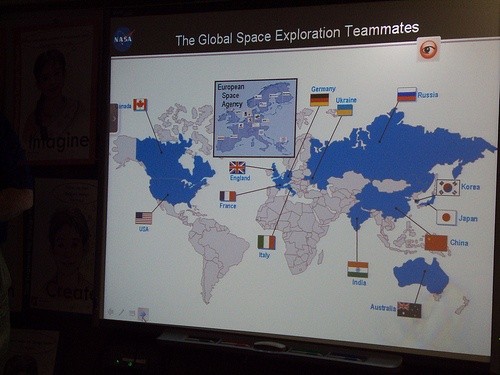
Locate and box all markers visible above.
[290,349,324,356]
[220,339,250,347]
[327,354,363,362]
[185,337,215,344]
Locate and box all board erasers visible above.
[253,340,288,353]
